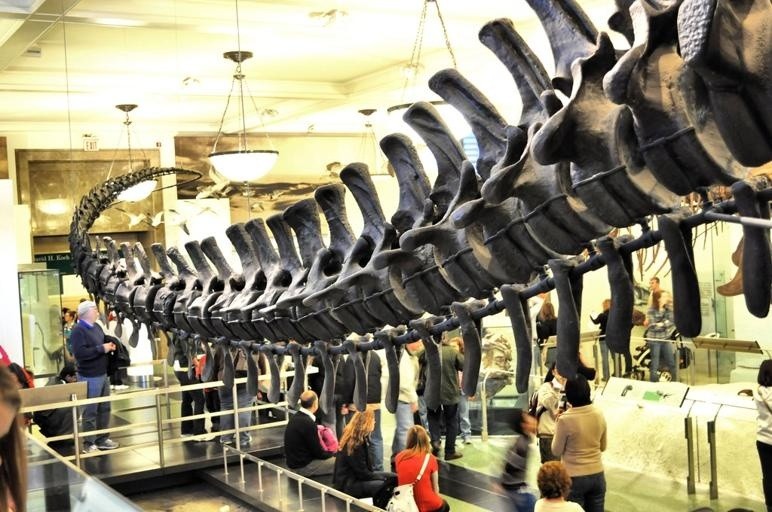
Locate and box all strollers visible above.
[628,312,697,382]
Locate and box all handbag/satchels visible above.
[386,484,419,512]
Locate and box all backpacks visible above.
[528,382,553,421]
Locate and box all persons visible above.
[283,390,336,477]
[332,405,397,508]
[494,410,540,512]
[645,276,674,321]
[648,290,677,384]
[171,318,475,458]
[32,365,81,447]
[533,457,586,511]
[395,423,451,511]
[49,298,151,391]
[0,364,28,512]
[70,301,120,454]
[534,303,557,348]
[753,359,772,511]
[551,375,609,512]
[588,299,617,381]
[529,361,570,463]
[613,325,635,379]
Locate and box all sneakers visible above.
[181,433,215,442]
[219,436,252,447]
[96,438,121,450]
[84,445,102,457]
[432,441,441,455]
[445,453,462,460]
[464,438,471,444]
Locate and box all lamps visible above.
[358,109,394,182]
[106,105,158,204]
[208,51,279,186]
[387,0,473,146]
